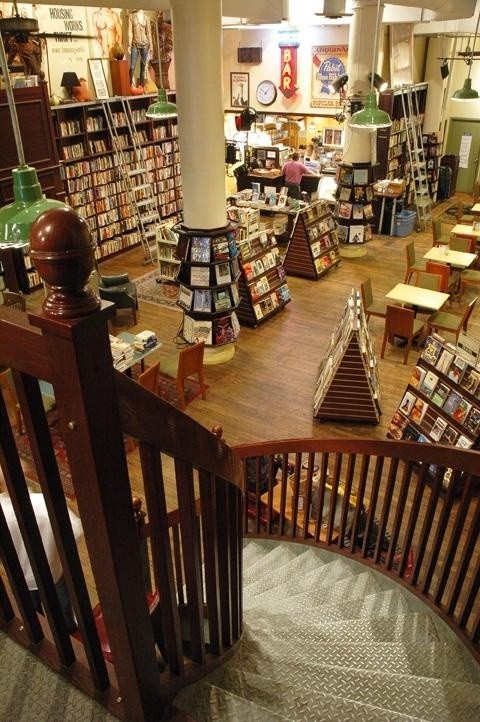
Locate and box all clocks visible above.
[257,80,277,106]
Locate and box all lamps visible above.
[145,19,177,118]
[0,34,71,249]
[328,0,392,128]
[451,13,480,102]
[61,72,81,103]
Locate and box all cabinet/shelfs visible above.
[377,82,428,209]
[387,334,480,500]
[168,223,242,346]
[232,229,295,331]
[51,92,176,263]
[228,146,332,243]
[314,289,381,424]
[158,222,238,284]
[282,198,342,282]
[333,164,374,245]
[0,72,66,294]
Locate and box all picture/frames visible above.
[230,72,250,107]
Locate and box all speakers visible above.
[238,46,263,63]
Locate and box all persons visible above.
[92,7,123,59]
[283,152,317,198]
[129,9,153,89]
[0,456,83,635]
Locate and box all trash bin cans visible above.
[394,210,416,237]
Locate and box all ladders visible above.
[401,84,433,233]
[101,98,163,266]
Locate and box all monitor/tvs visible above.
[265,150,277,159]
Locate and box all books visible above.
[59,114,184,260]
[109,329,157,372]
[335,166,375,243]
[0,261,10,306]
[312,289,385,418]
[22,247,44,287]
[388,118,439,204]
[158,182,341,347]
[1,73,42,89]
[387,336,480,491]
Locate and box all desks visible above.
[260,469,370,542]
[38,332,161,398]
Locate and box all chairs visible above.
[247,461,295,531]
[0,368,56,436]
[139,361,160,394]
[361,201,480,365]
[97,273,137,324]
[159,341,206,410]
